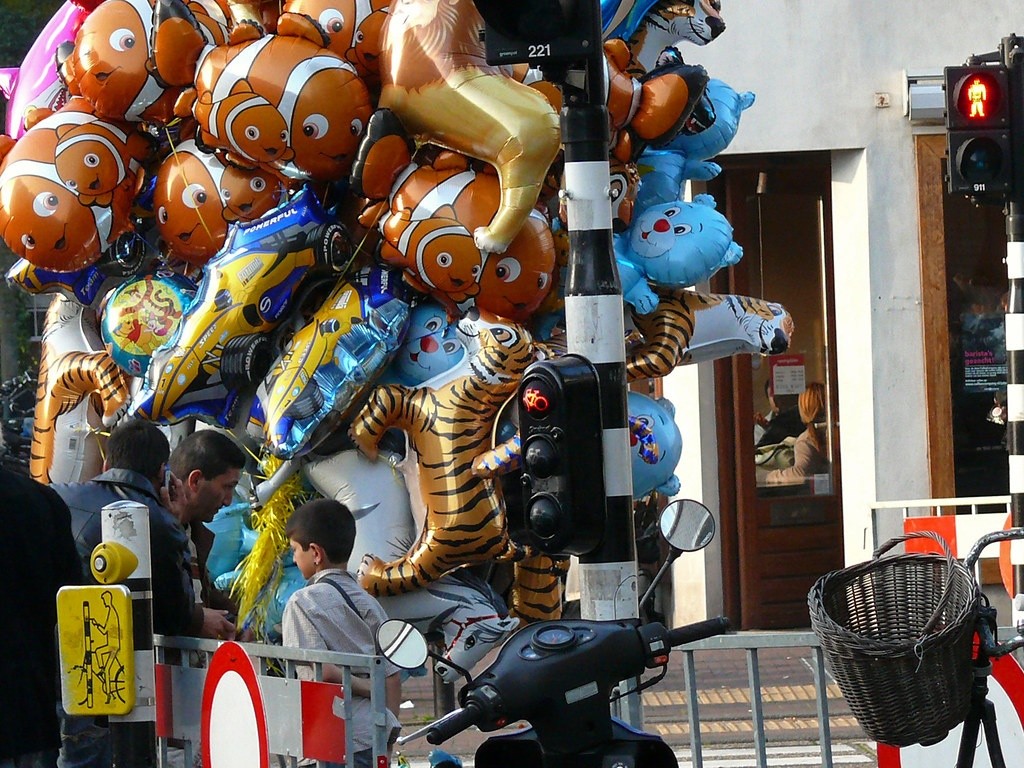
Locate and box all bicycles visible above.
[808,526,1024,768]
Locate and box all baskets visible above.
[807,530,983,747]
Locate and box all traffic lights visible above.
[944,66,1013,199]
[517,354,609,559]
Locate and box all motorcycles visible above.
[375,499,734,768]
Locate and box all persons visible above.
[282,498,402,768]
[0,467,82,768]
[47,419,245,768]
[754,379,827,483]
[946,256,1011,359]
